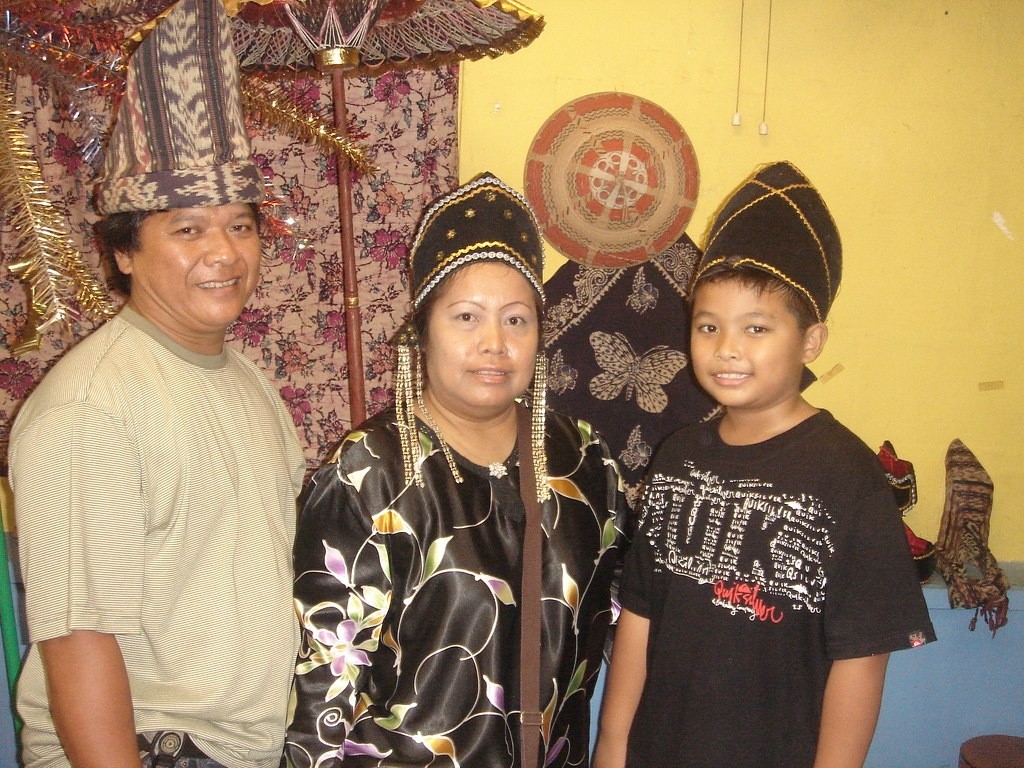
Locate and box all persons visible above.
[592,228,936,768]
[8,153,308,768]
[282,227,629,767]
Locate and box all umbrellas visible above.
[119,0,546,430]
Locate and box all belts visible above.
[137,731,208,759]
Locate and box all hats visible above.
[93,0,264,217]
[408,171,546,317]
[685,159,842,326]
